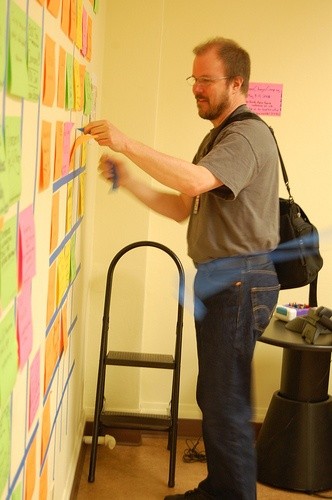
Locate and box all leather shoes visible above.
[164,487,217,500]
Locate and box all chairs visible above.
[86,240,185,488]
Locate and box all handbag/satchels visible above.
[267,196,323,291]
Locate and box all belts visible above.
[197,251,273,271]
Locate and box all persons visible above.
[81,33,284,500]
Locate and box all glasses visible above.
[186,75,241,87]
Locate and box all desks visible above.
[253,312,332,495]
